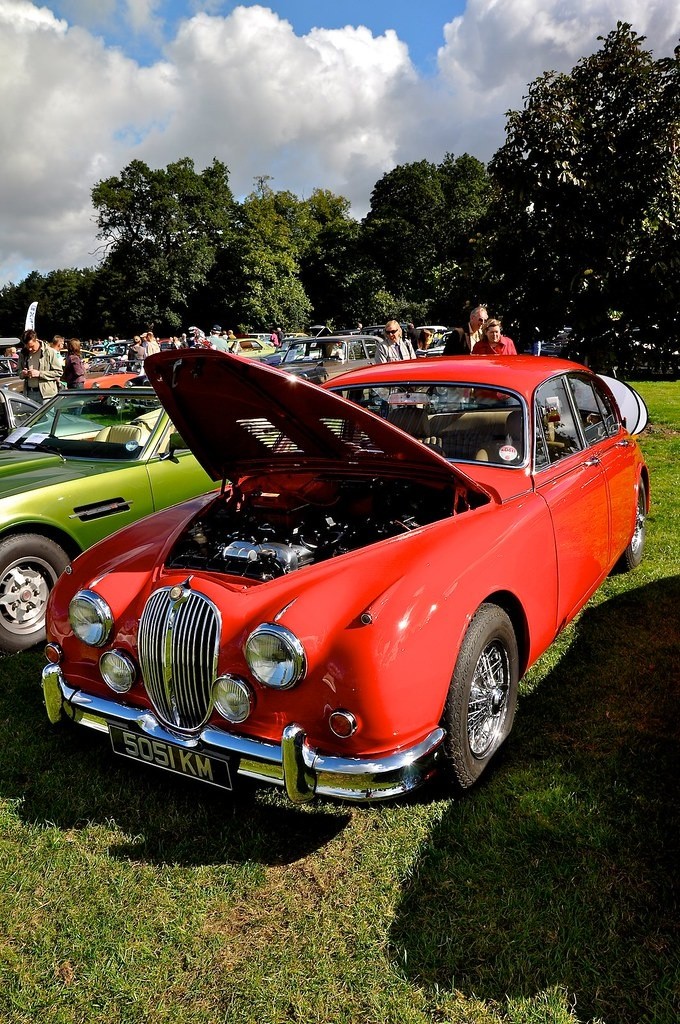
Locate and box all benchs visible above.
[427,412,572,464]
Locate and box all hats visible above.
[211,325,222,333]
[133,336,141,342]
[140,333,147,337]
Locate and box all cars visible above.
[0,322,453,450]
[41,347,650,805]
[0,387,340,657]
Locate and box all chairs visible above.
[476,410,524,465]
[386,408,446,458]
[94,424,151,448]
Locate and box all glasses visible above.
[473,315,487,323]
[385,329,398,335]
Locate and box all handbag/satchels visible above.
[60,359,68,382]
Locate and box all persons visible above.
[270,328,284,348]
[375,306,517,364]
[0,325,236,406]
[357,320,363,330]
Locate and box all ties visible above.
[395,343,403,360]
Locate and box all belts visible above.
[27,387,39,392]
